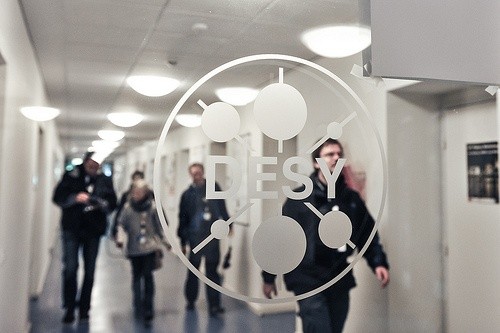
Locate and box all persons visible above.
[178,163,235,316]
[52,150,116,324]
[112,171,172,322]
[263,138,390,333]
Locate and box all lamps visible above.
[175,114,202,128]
[300,23,372,58]
[127,75,180,98]
[20,106,60,122]
[215,87,260,107]
[98,131,125,142]
[107,113,144,127]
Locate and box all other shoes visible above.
[135,308,154,321]
[186,301,195,312]
[79,309,89,323]
[61,310,75,324]
[208,307,226,318]
